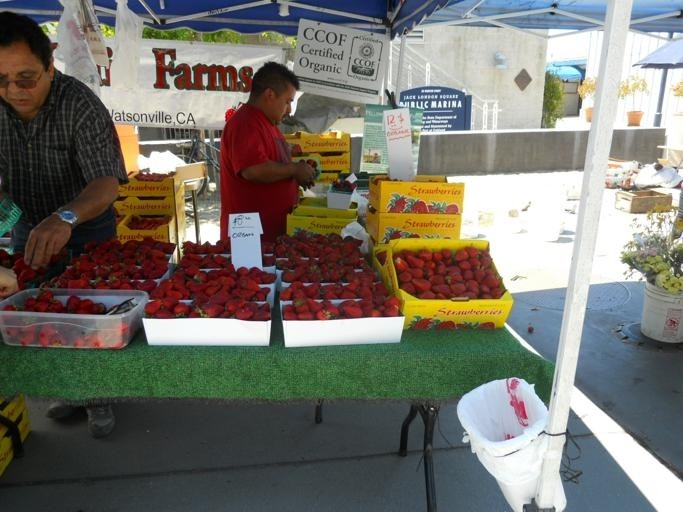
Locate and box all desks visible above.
[1,263,556,512]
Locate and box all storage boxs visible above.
[50,133,512,346]
[1,289,148,348]
[614,190,672,214]
[0,393,29,473]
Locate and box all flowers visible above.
[619,202,682,296]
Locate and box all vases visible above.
[639,281,682,344]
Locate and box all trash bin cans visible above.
[456,376,568,512]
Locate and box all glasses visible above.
[0,69,44,88]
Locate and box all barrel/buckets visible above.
[639,282,682,343]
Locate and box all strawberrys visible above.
[1,174,505,350]
[225,105,238,124]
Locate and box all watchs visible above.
[51,206,77,233]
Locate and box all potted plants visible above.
[576,75,597,122]
[618,73,649,126]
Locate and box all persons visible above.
[0,11,128,436]
[218,61,316,245]
[0,265,20,298]
